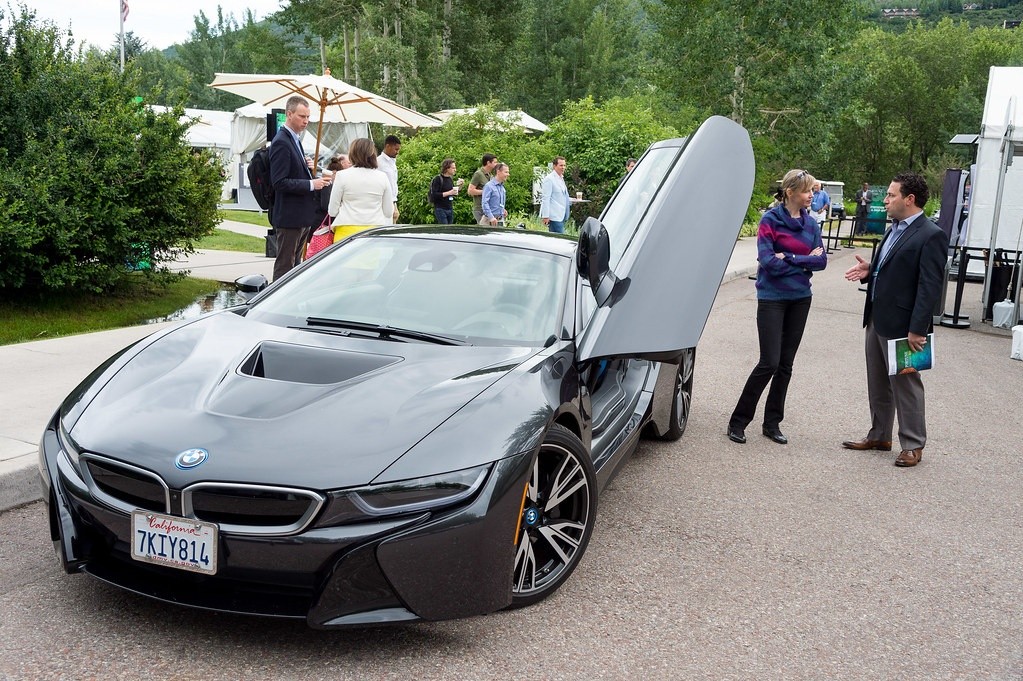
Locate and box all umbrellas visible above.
[428,105,550,137]
[206,67,445,177]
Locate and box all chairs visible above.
[378,250,474,328]
[491,283,552,321]
[517,258,564,335]
[477,252,513,282]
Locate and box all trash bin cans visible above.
[866,193,887,235]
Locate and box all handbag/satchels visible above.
[303,213,334,259]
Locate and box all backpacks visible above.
[428,174,445,203]
[246,141,273,209]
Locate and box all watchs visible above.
[821,208,824,211]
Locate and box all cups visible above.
[322,170,332,186]
[576,192,582,201]
[452,187,459,195]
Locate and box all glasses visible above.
[790,170,810,187]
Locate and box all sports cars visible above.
[36,115,757,628]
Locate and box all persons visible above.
[618,157,638,189]
[301,135,402,260]
[468,151,510,227]
[854,182,873,236]
[840,171,951,468]
[539,156,576,233]
[266,96,332,282]
[725,169,829,444]
[430,159,466,225]
[808,181,829,231]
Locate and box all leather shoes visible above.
[726,426,748,443]
[761,428,788,444]
[843,437,895,451]
[893,448,925,467]
